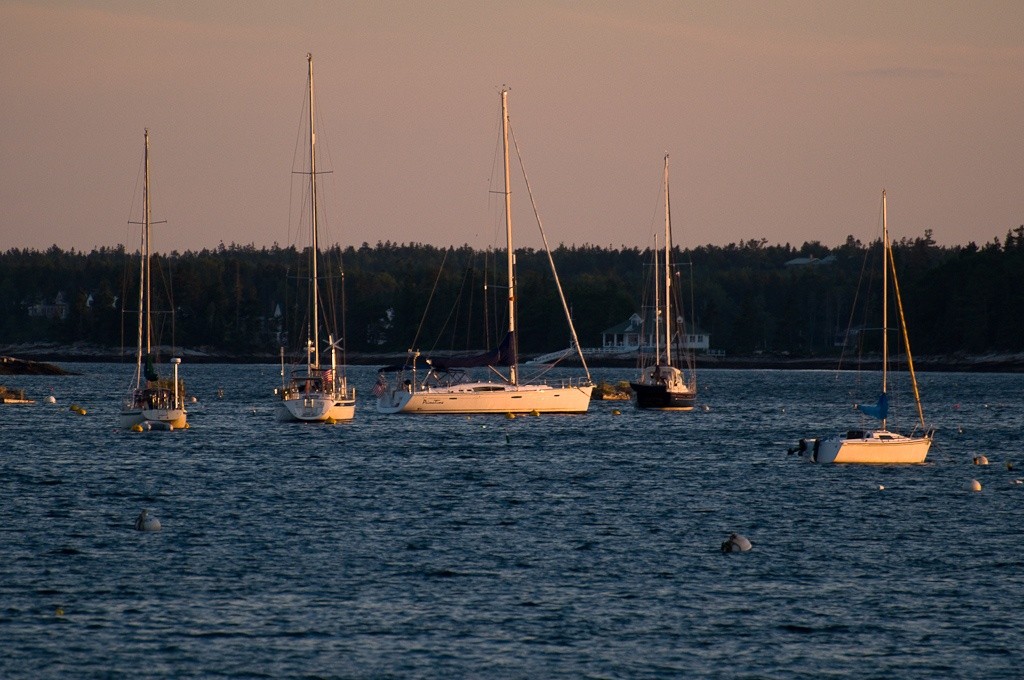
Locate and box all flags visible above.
[321,368,332,383]
[372,380,385,398]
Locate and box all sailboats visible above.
[117,128,189,429]
[376,83,598,416]
[272,51,357,422]
[799,188,937,465]
[628,147,698,412]
